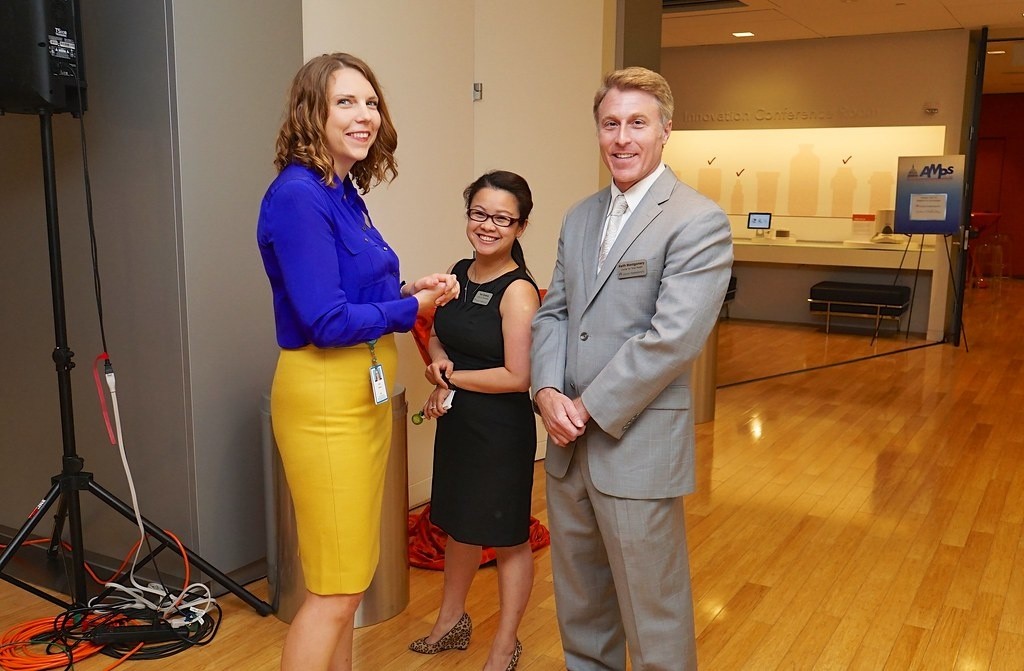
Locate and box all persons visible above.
[408,168,544,670]
[527,64,737,670]
[252,52,463,670]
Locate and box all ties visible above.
[598,196,628,270]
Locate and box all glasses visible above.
[467,208,519,228]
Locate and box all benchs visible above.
[724,276,736,319]
[807,281,912,338]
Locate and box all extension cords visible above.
[90,623,189,645]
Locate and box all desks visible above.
[726,214,960,341]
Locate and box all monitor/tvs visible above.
[747,212,771,236]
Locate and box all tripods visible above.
[0,118,275,617]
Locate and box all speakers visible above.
[0,0,88,117]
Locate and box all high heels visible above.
[483,638,523,671]
[409,612,472,654]
